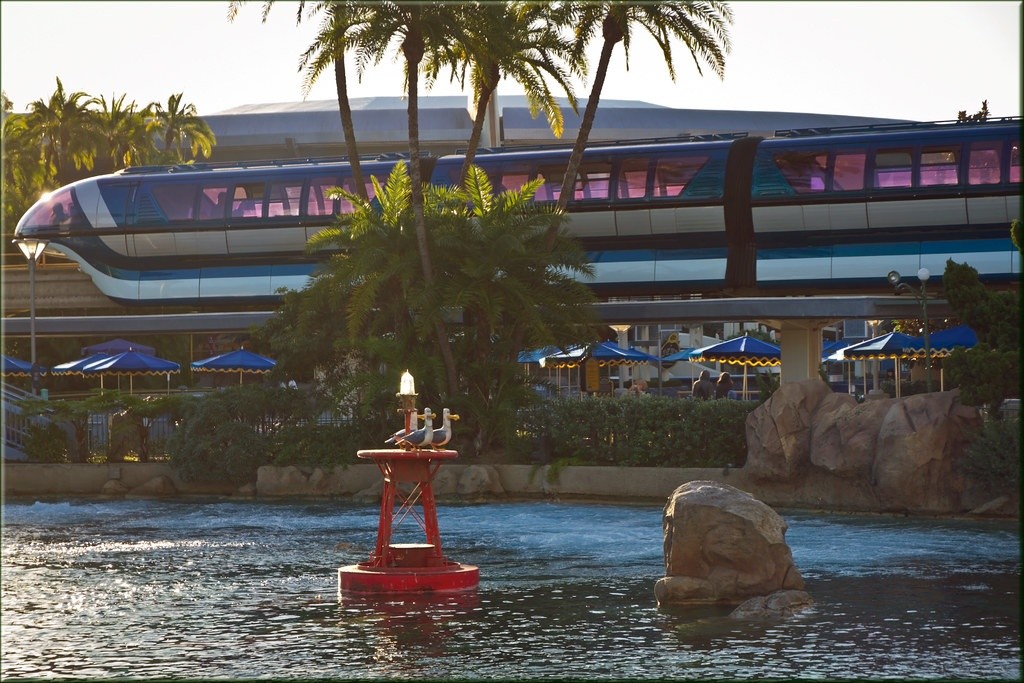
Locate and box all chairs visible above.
[233,199,255,216]
[212,192,226,218]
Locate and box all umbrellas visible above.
[689,332,782,400]
[538,341,648,368]
[50,346,180,395]
[82,338,156,389]
[190,346,276,383]
[843,323,977,396]
[0,354,47,376]
[663,347,696,383]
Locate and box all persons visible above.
[716,372,734,398]
[691,369,715,400]
[48,202,67,224]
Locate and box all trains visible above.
[7,118,1024,309]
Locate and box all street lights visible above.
[12,237,50,394]
[888,268,933,393]
